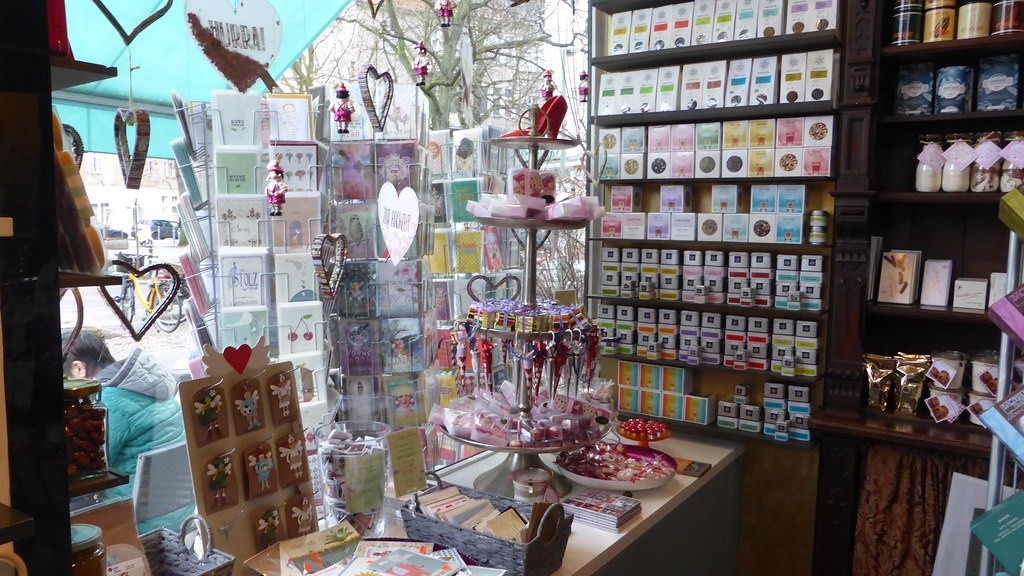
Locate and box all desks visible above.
[243,427,747,576]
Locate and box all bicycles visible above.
[111,251,189,333]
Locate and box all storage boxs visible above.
[595,0,839,426]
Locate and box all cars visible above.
[96,222,129,241]
[130,218,181,241]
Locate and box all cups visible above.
[314,420,390,539]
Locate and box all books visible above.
[560,489,642,534]
[367,547,464,576]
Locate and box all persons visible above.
[540,70,555,101]
[579,71,588,102]
[59,329,197,538]
[411,42,430,86]
[328,83,354,134]
[265,161,288,215]
[434,0,457,27]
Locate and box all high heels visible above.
[500,95,567,139]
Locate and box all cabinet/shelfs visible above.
[808,0,1024,462]
[0,0,130,576]
[427,97,621,496]
[581,0,848,452]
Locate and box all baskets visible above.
[139,515,236,576]
[400,471,574,576]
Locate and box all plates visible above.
[554,442,678,491]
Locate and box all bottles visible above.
[511,467,554,504]
[916,132,1024,192]
